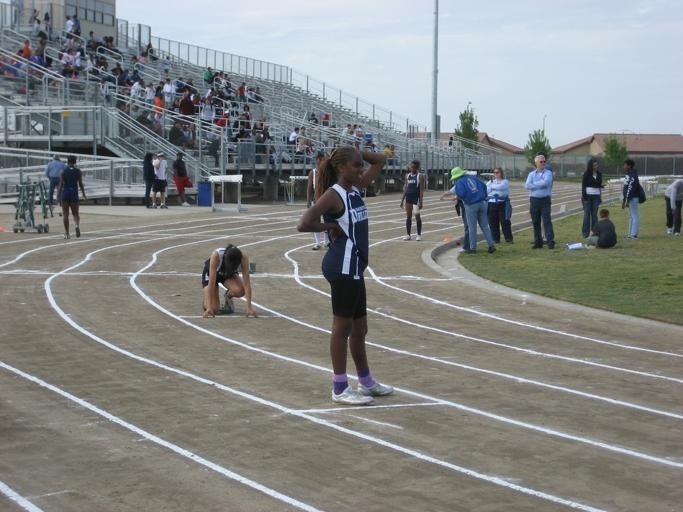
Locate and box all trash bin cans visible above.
[224,182,237,203]
[262,176,278,201]
[196,181,211,207]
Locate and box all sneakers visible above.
[223,293,235,312]
[61,225,81,239]
[144,202,192,209]
[666,228,681,239]
[416,234,422,241]
[402,235,411,240]
[331,384,374,405]
[583,233,639,250]
[311,241,331,250]
[459,239,555,255]
[357,380,394,396]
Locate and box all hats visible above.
[448,166,468,181]
[157,151,165,157]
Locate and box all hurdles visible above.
[287,176,310,206]
[480,173,495,177]
[608,176,659,199]
[208,175,248,213]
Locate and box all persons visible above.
[484,167,513,243]
[664,178,683,237]
[583,209,617,250]
[524,151,552,245]
[294,144,396,406]
[620,160,641,240]
[199,244,259,319]
[579,158,603,239]
[523,154,555,250]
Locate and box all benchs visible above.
[268,119,444,170]
[0,25,98,67]
[222,72,374,122]
[108,49,219,96]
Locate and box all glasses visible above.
[494,171,500,174]
[539,160,546,163]
